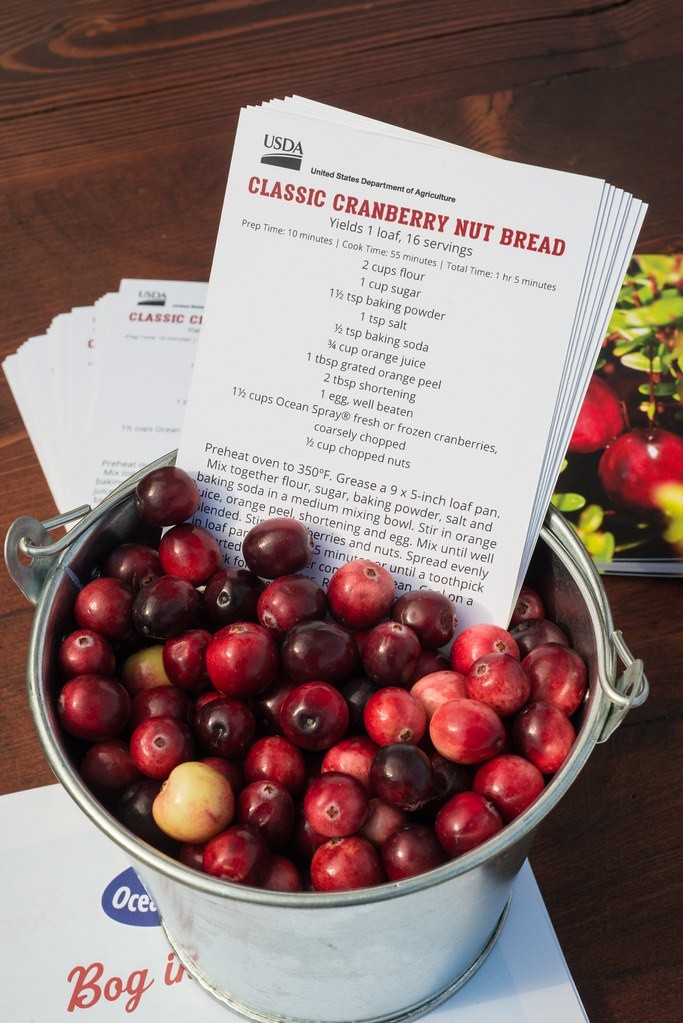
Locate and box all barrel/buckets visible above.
[4,450,649,1022]
